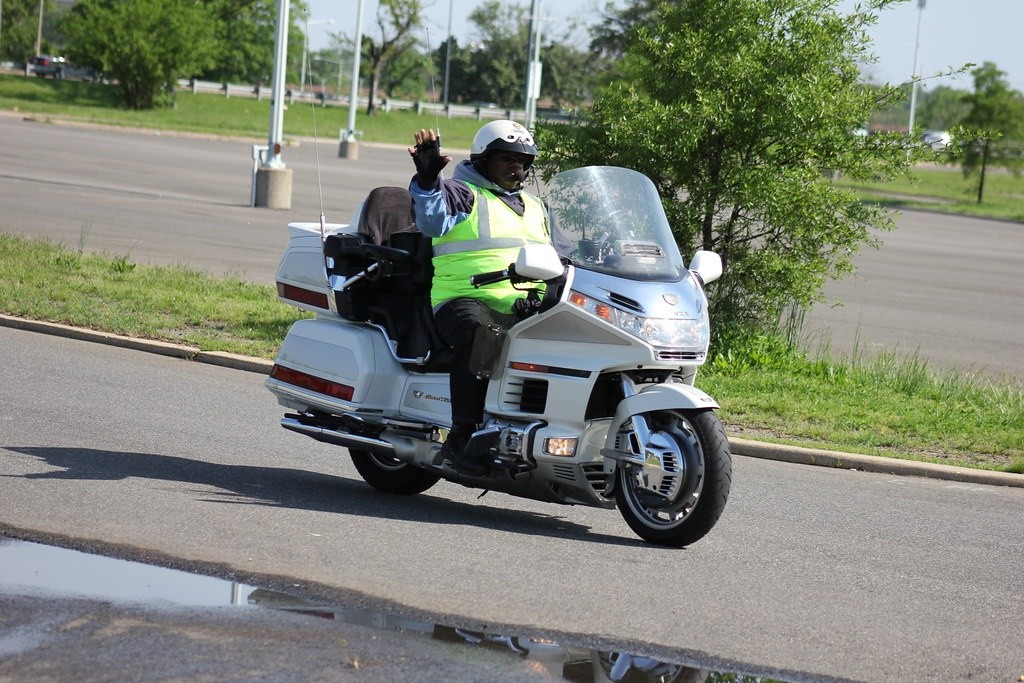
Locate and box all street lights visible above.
[905,0,925,156]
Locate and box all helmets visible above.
[470,119,538,162]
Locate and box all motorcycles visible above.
[266,166,736,548]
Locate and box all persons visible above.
[407,126,576,477]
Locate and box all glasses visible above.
[487,153,528,164]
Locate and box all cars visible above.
[26,52,103,82]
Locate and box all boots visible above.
[442,377,490,476]
